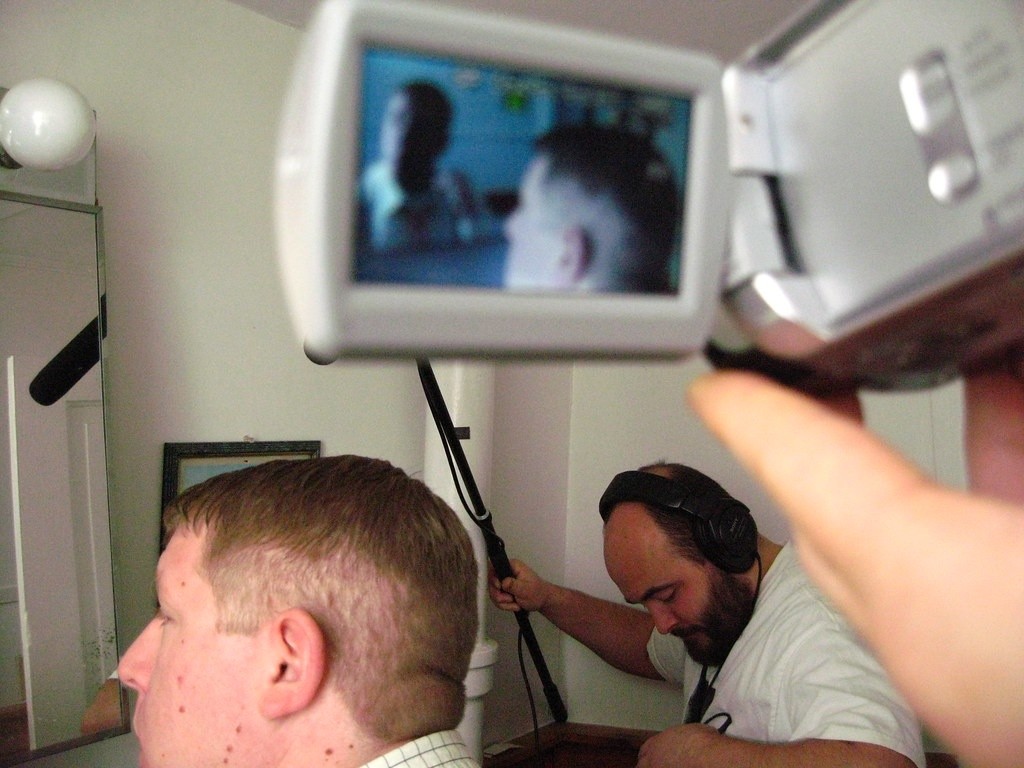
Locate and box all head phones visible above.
[597,470,759,574]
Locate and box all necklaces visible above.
[700,547,761,687]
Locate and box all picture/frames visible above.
[160,440,321,555]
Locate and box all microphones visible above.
[29,317,99,406]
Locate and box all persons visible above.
[690,342,1024,768]
[80,667,123,733]
[113,454,485,768]
[485,460,929,768]
[348,67,685,300]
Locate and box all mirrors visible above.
[0,190,132,768]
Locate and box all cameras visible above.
[278,0,1024,395]
